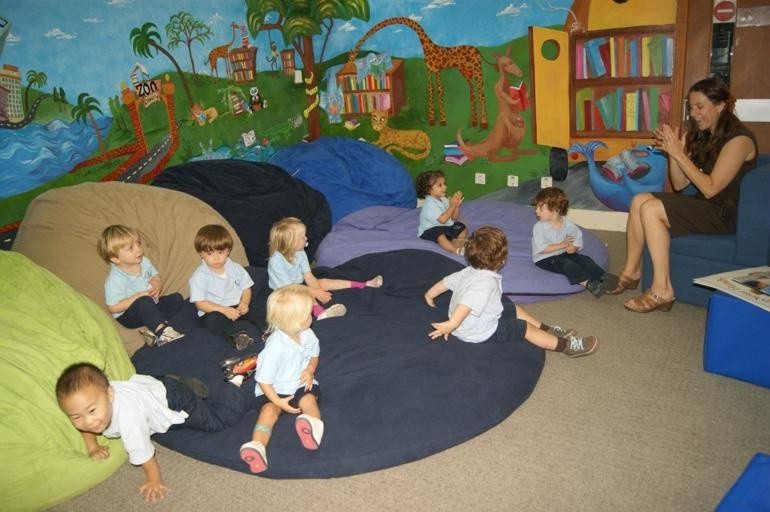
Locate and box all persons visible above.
[265,216,385,322]
[413,169,468,257]
[187,224,273,350]
[55,351,259,505]
[530,186,620,299]
[603,75,759,314]
[424,226,599,359]
[94,224,187,349]
[237,282,325,474]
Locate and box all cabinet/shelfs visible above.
[526,0,689,180]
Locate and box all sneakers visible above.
[138,329,184,347]
[166,373,210,401]
[455,245,465,258]
[219,352,260,381]
[562,333,599,358]
[235,333,254,351]
[317,304,347,321]
[367,275,382,288]
[588,272,619,298]
[547,324,579,339]
[239,441,268,473]
[295,414,324,450]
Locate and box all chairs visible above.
[641,151,770,308]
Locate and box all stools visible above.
[713,453,770,512]
[701,290,770,387]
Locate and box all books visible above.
[338,74,392,114]
[443,143,469,156]
[507,80,529,112]
[280,52,294,75]
[228,50,255,117]
[691,261,770,314]
[443,154,468,167]
[572,33,671,134]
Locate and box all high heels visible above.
[605,269,639,297]
[623,288,676,313]
